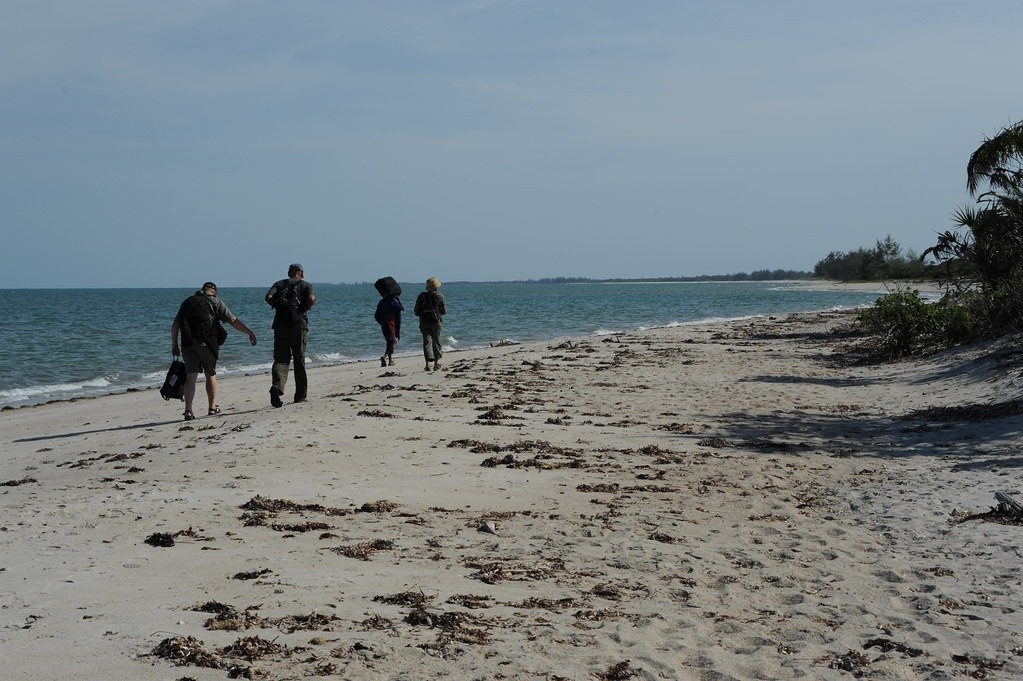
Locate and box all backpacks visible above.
[160,356,186,403]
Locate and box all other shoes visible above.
[388,361,395,366]
[381,357,386,367]
[433,364,441,371]
[424,366,430,371]
[294,395,308,403]
[270,387,282,408]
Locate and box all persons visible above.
[264,263,316,407]
[170,281,257,420]
[374,295,404,366]
[414,276,447,371]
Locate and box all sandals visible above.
[208,404,221,415]
[182,411,195,420]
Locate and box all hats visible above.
[426,277,442,290]
[290,263,304,279]
[204,282,217,290]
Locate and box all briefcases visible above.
[374,276,401,298]
